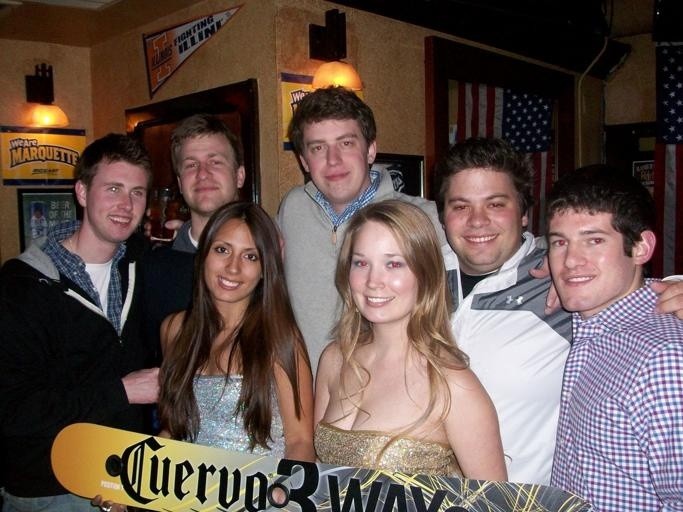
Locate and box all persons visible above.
[546,164,683,512]
[144,113,283,360]
[1,132,163,512]
[31,206,48,238]
[431,136,683,486]
[309,199,508,481]
[91,201,315,512]
[164,85,562,412]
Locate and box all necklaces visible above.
[68,238,109,297]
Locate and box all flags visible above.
[653,45,683,280]
[456,83,553,238]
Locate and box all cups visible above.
[147,181,184,242]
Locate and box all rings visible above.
[101,506,113,512]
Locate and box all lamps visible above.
[25,62,68,127]
[308,9,364,91]
[141,187,188,244]
